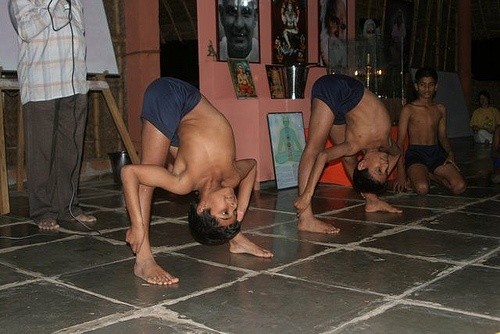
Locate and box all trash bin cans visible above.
[108,151,139,182]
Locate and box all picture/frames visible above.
[214,0,261,64]
[317,0,348,68]
[267,112,308,191]
[0,1,120,77]
[271,0,309,64]
[226,58,258,100]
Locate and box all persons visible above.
[392,67,466,194]
[318,1,347,65]
[7,0,96,230]
[470,91,500,183]
[294,74,403,234]
[120,77,273,285]
[217,0,259,62]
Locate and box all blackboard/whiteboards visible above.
[0,0,120,78]
